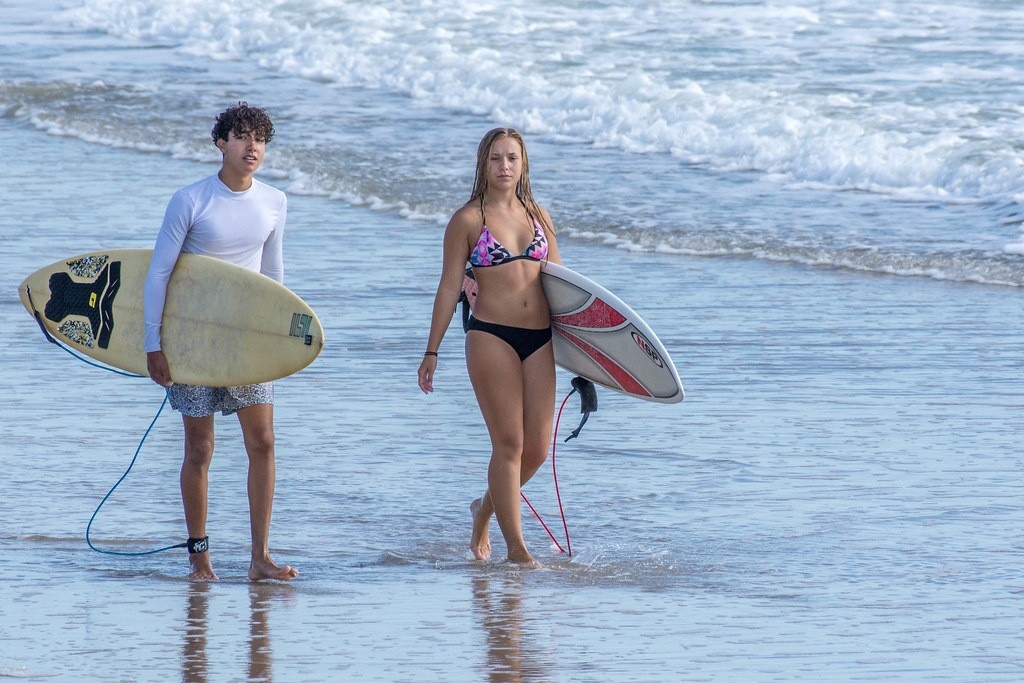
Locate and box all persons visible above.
[418,128,564,566]
[142,106,299,581]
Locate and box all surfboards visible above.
[17,248,323,388]
[458,256,686,405]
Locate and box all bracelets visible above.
[424,352,438,357]
